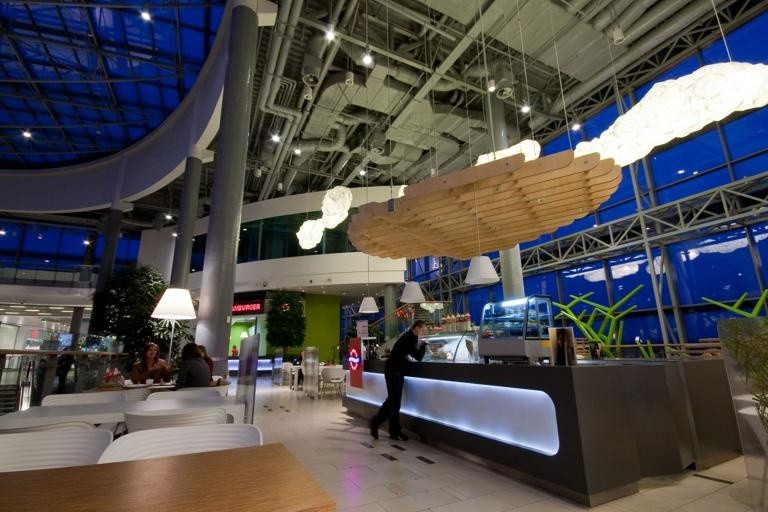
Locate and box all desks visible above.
[4,442,335,508]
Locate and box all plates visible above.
[122,384,146,388]
[161,382,171,386]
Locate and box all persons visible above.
[56,351,73,390]
[370,321,429,441]
[197,345,214,384]
[131,343,171,384]
[176,343,211,388]
[231,346,238,359]
[293,351,304,385]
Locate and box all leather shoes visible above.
[370,417,379,440]
[389,432,409,441]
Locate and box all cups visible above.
[145,379,154,386]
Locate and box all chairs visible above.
[1,373,263,472]
[277,359,348,400]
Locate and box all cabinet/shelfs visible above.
[476,294,555,367]
[408,331,482,365]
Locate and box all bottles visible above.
[374,344,381,360]
[442,311,472,332]
[555,329,570,366]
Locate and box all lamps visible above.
[294,1,767,253]
[356,246,382,314]
[465,182,501,288]
[148,286,199,368]
[397,257,428,303]
